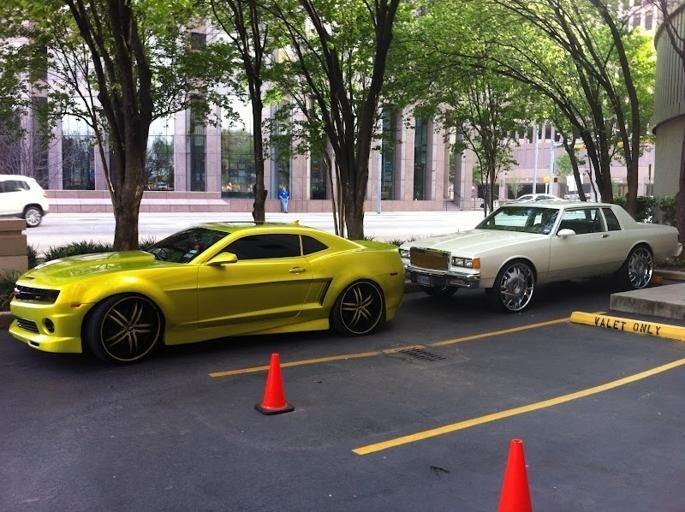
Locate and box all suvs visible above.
[0,174,49,228]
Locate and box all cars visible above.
[503,194,569,216]
[145,181,173,191]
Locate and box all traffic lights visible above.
[554,176,558,183]
[613,155,621,159]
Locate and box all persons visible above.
[278,185,290,213]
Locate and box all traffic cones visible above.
[254,353,296,416]
[498,439,533,512]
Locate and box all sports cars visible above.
[399,198,683,314]
[8,219,406,366]
